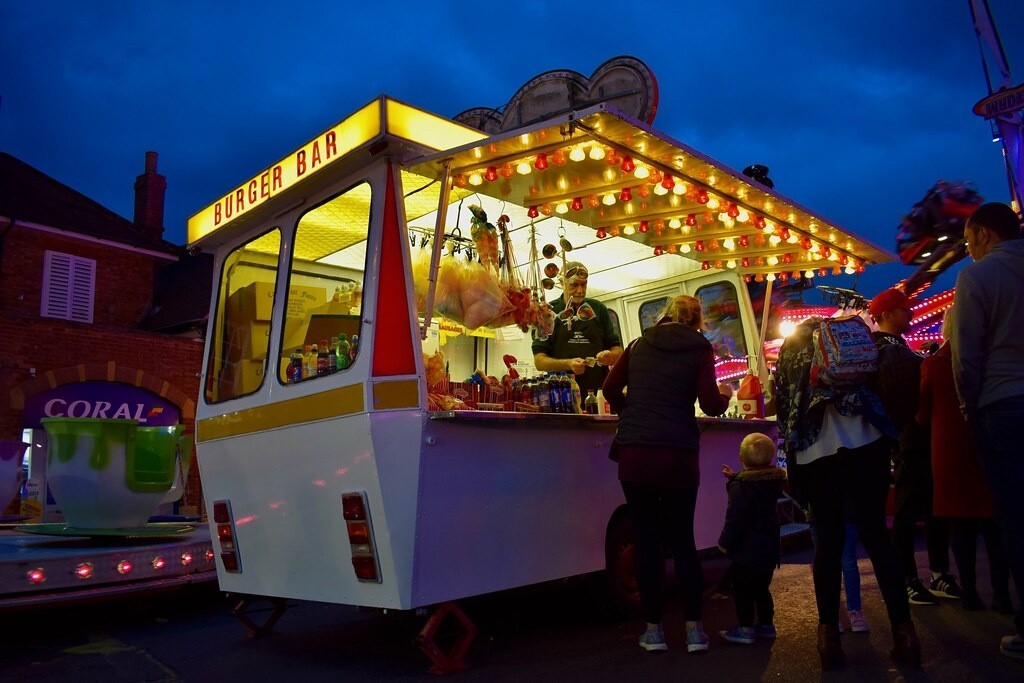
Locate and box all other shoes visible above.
[718,627,756,643]
[757,626,777,638]
[1000,634,1024,658]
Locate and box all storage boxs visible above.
[219,283,327,401]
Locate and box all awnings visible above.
[404,104,898,376]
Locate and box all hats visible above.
[870,290,923,323]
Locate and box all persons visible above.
[776,315,922,669]
[949,203,1024,657]
[602,295,732,653]
[530,261,623,410]
[869,291,1012,613]
[717,432,787,644]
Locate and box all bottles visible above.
[286,333,358,382]
[513,369,582,413]
[597,390,610,414]
[585,389,599,413]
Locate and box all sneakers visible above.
[847,609,866,631]
[838,622,846,631]
[907,578,939,604]
[638,627,665,651]
[687,628,709,651]
[929,572,959,598]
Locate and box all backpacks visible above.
[811,313,879,392]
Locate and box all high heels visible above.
[818,624,846,671]
[889,621,922,670]
